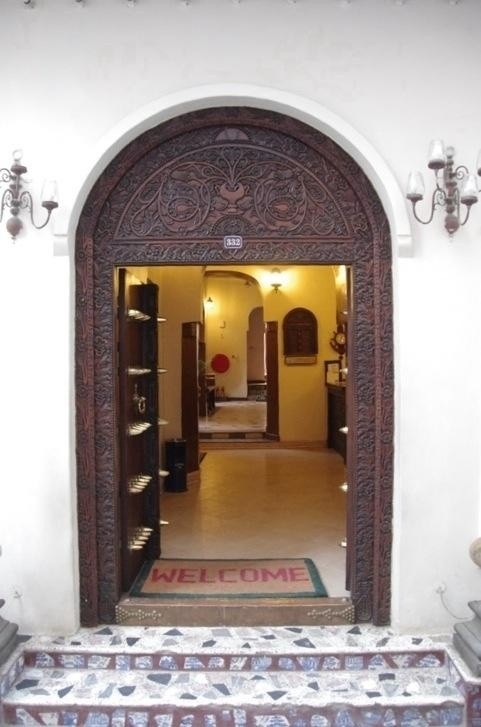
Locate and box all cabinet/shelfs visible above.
[119,268,160,590]
[182,320,216,473]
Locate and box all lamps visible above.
[404,138,481,233]
[204,297,213,312]
[269,267,282,295]
[0,148,58,239]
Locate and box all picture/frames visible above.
[324,360,342,385]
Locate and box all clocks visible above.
[329,325,349,354]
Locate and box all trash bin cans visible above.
[164,439,187,492]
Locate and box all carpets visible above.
[130,556,334,598]
